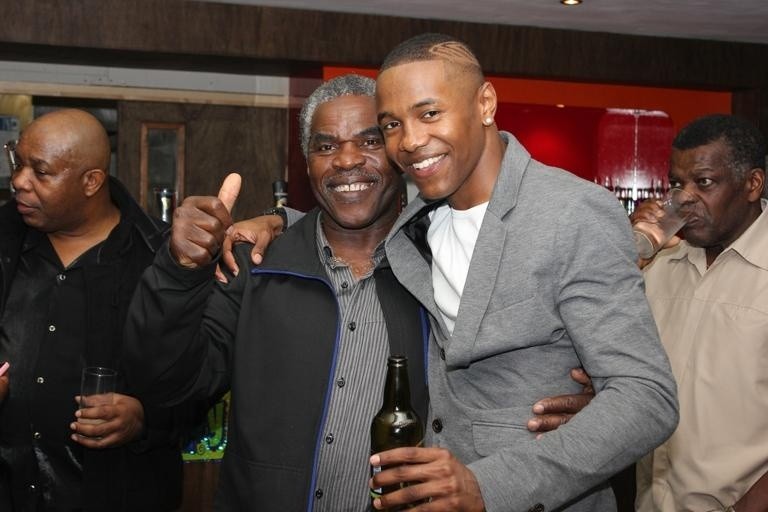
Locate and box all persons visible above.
[628,113,768,512]
[122,71,595,512]
[214,31,680,512]
[0,107,170,512]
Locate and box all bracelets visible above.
[727,506,735,512]
[264,208,289,232]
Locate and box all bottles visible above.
[369,354,425,510]
[593,173,681,217]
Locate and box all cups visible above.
[633,189,700,259]
[78,364,119,440]
